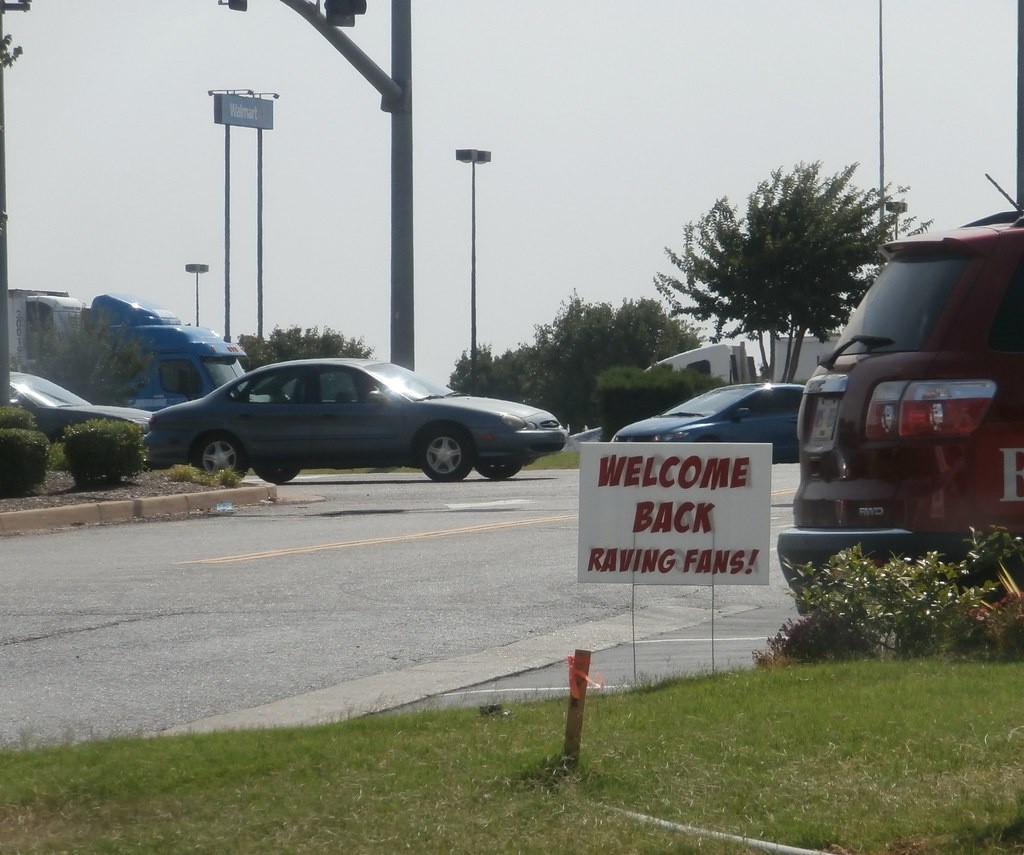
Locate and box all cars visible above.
[10,371,156,462]
[143,357,569,483]
[609,383,805,464]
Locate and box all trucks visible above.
[642,337,844,385]
[6,288,247,412]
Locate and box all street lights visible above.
[886,202,907,240]
[455,150,491,377]
[186,264,208,327]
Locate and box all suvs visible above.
[777,173,1024,617]
